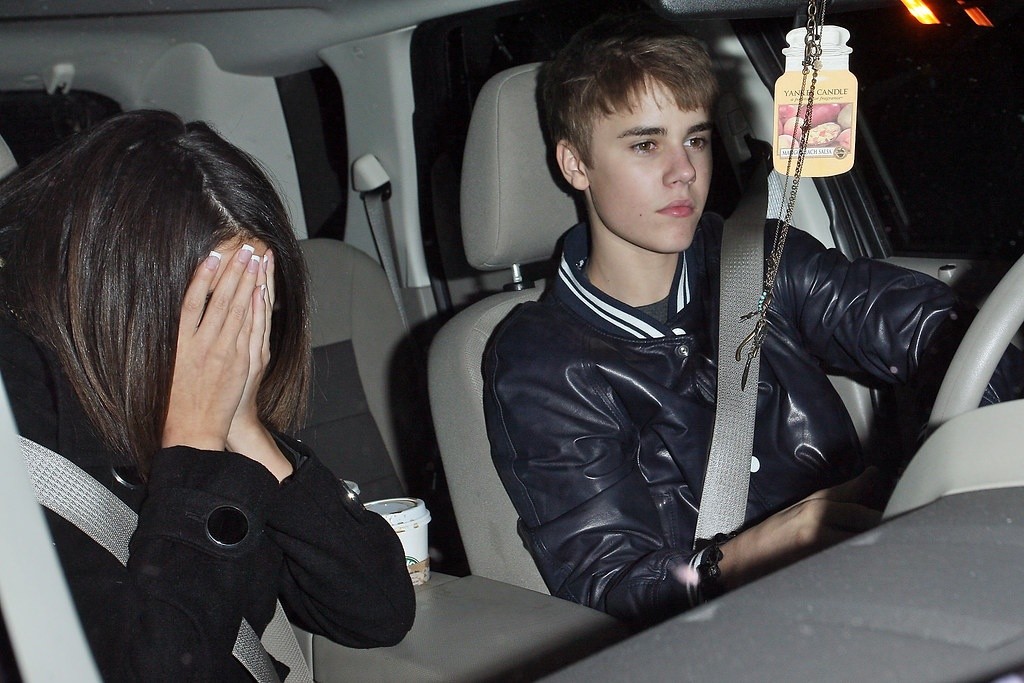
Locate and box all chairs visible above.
[427,60,584,596]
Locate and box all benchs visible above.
[285,238,469,575]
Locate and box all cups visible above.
[339,478,361,498]
[361,498,432,586]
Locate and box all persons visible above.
[481,10,1023,629]
[0,107,417,683]
